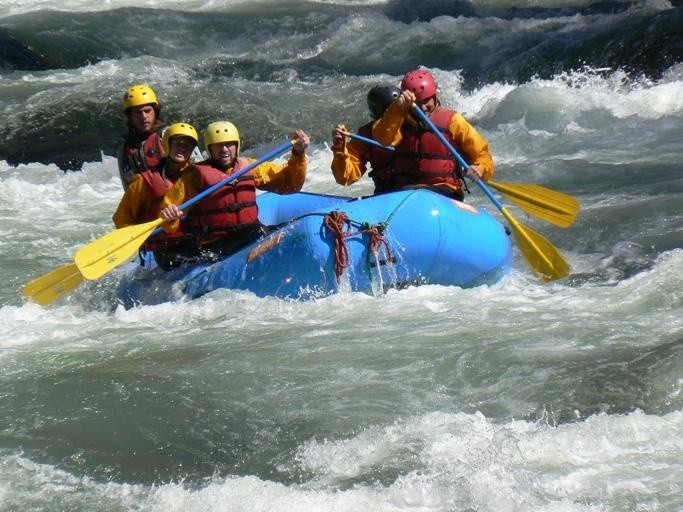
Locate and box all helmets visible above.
[402,70,437,107]
[204,120,240,160]
[162,123,199,158]
[123,84,158,110]
[368,83,402,118]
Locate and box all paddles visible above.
[75,137,303,281]
[342,131,580,228]
[411,101,570,284]
[21,214,191,306]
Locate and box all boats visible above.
[112,188,514,310]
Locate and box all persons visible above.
[371,69,493,203]
[112,123,216,273]
[116,86,205,193]
[155,122,308,260]
[329,84,408,195]
